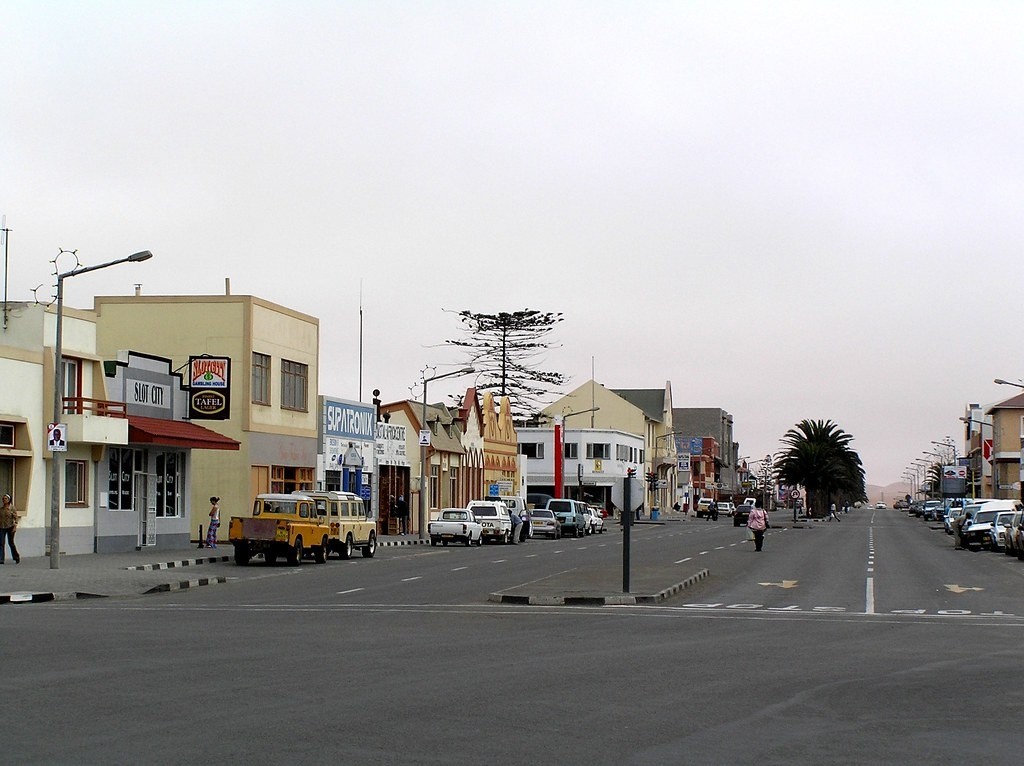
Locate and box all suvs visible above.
[288,490,378,559]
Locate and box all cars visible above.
[892,497,1024,562]
[695,496,757,527]
[529,508,562,540]
[874,502,888,509]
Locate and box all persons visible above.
[674,502,680,511]
[508,510,523,545]
[953,513,972,550]
[205,497,220,548]
[396,497,407,535]
[0,493,21,565]
[747,502,768,552]
[50,429,64,446]
[798,503,803,514]
[829,501,840,522]
[706,499,718,521]
[845,500,848,513]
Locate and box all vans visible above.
[526,493,604,538]
[461,501,512,545]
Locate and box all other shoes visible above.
[755,548,761,552]
[0,561,5,564]
[15,554,19,563]
[206,545,211,547]
[512,542,518,545]
[838,519,841,522]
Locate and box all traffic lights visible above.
[646,472,652,482]
[649,483,654,491]
[653,483,659,491]
[652,473,659,482]
[628,467,637,479]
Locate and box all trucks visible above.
[484,494,533,539]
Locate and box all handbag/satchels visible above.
[831,512,834,518]
[763,510,770,528]
[217,523,220,527]
[746,526,755,541]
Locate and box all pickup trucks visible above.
[428,508,484,548]
[227,492,330,568]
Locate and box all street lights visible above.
[654,431,683,506]
[959,417,996,499]
[731,456,750,503]
[562,406,601,499]
[418,366,478,544]
[910,462,927,500]
[901,476,913,498]
[905,466,920,500]
[930,440,957,498]
[699,445,722,500]
[902,472,917,500]
[747,459,764,498]
[915,458,935,499]
[923,451,943,500]
[48,249,154,569]
